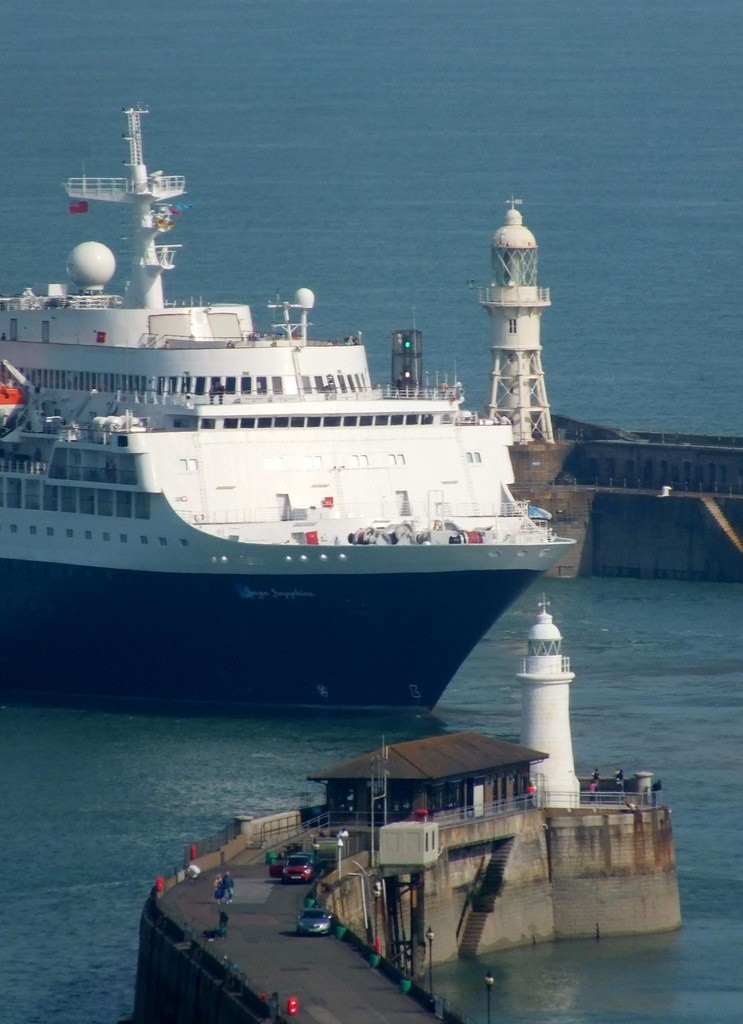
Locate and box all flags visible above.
[66,200,88,216]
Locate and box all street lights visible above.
[373,885,381,954]
[337,839,343,881]
[485,972,494,1023]
[311,838,320,883]
[426,925,434,1014]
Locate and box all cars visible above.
[270,856,313,883]
[296,908,332,937]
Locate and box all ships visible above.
[0,110,577,704]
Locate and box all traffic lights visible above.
[402,335,411,350]
[404,366,411,382]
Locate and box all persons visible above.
[337,827,350,840]
[652,778,664,807]
[225,327,411,396]
[208,384,216,405]
[510,418,513,425]
[526,782,539,805]
[611,767,621,781]
[498,414,513,427]
[221,871,235,904]
[531,428,543,440]
[216,909,229,936]
[316,828,330,839]
[185,864,203,880]
[615,769,625,792]
[212,872,224,904]
[591,766,599,789]
[588,778,598,802]
[217,384,227,405]
[438,382,450,399]
